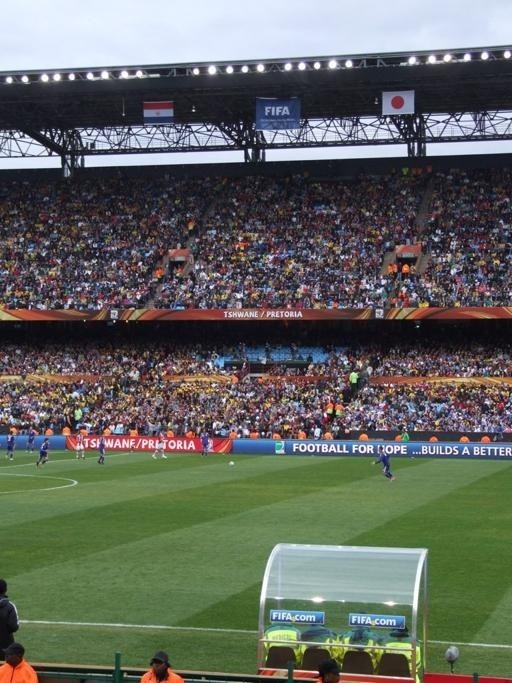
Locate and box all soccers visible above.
[228,460,235,465]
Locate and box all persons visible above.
[300,613,343,670]
[378,616,424,683]
[0,643,38,682]
[0,577,20,661]
[141,650,185,683]
[317,658,341,682]
[340,618,382,675]
[263,611,301,670]
[1,168,510,480]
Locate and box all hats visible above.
[151,651,169,662]
[2,643,26,655]
[318,659,342,676]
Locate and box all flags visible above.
[255,94,302,129]
[141,98,174,125]
[381,89,416,116]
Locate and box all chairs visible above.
[302,648,330,670]
[266,646,296,669]
[377,652,411,676]
[341,651,374,674]
